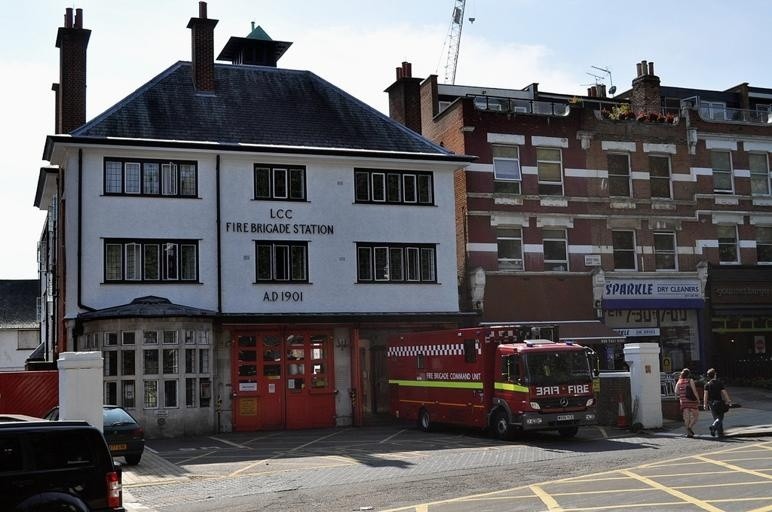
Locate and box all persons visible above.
[703,368,732,438]
[673,368,701,438]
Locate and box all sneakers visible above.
[710,425,715,436]
[688,428,694,435]
[719,436,728,440]
[687,435,693,438]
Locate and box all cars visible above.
[40,403,145,466]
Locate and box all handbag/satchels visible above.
[685,384,697,402]
[708,400,730,414]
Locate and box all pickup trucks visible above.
[1,411,129,510]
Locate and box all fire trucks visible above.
[387,324,601,438]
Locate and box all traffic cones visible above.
[612,393,632,430]
